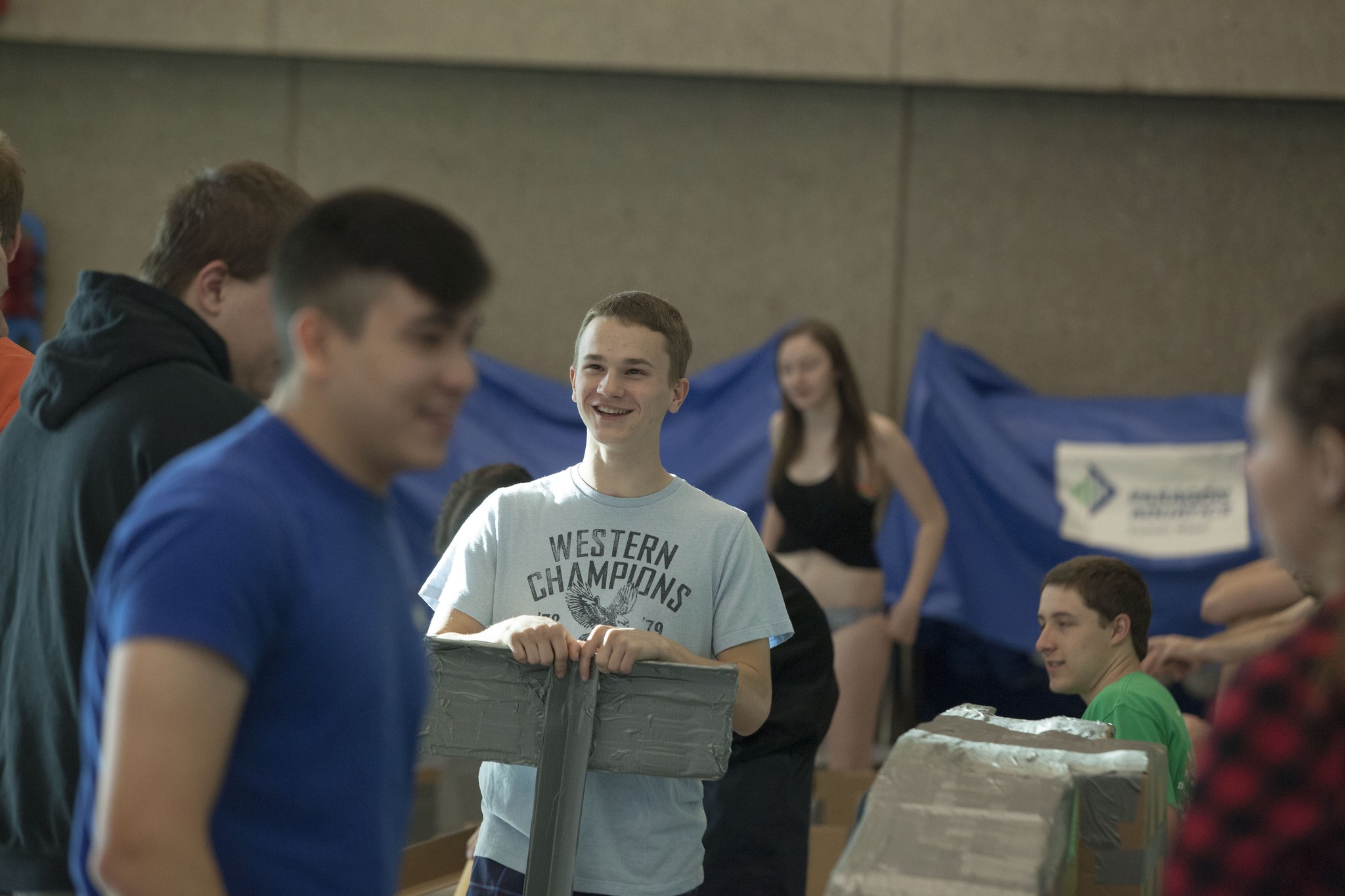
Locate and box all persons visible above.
[752,317,947,886]
[1034,555,1200,875]
[1133,296,1345,896]
[0,163,322,895]
[0,132,47,434]
[428,463,837,895]
[69,188,496,896]
[417,290,797,896]
